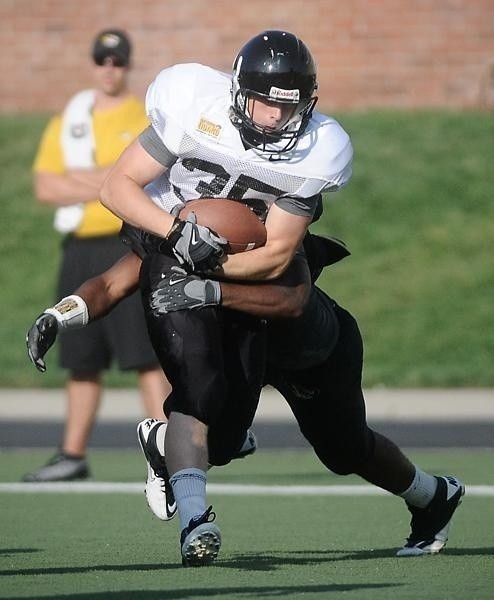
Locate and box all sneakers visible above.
[397,474,465,556]
[180,516,221,564]
[22,452,87,482]
[240,429,258,457]
[137,417,180,521]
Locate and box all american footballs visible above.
[176,198,267,252]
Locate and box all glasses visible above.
[94,55,125,68]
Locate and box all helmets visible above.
[229,30,318,153]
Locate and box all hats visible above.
[92,30,128,54]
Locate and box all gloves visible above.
[162,220,229,273]
[27,312,58,372]
[150,266,221,316]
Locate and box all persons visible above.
[97,26,355,566]
[24,192,467,559]
[21,24,171,483]
